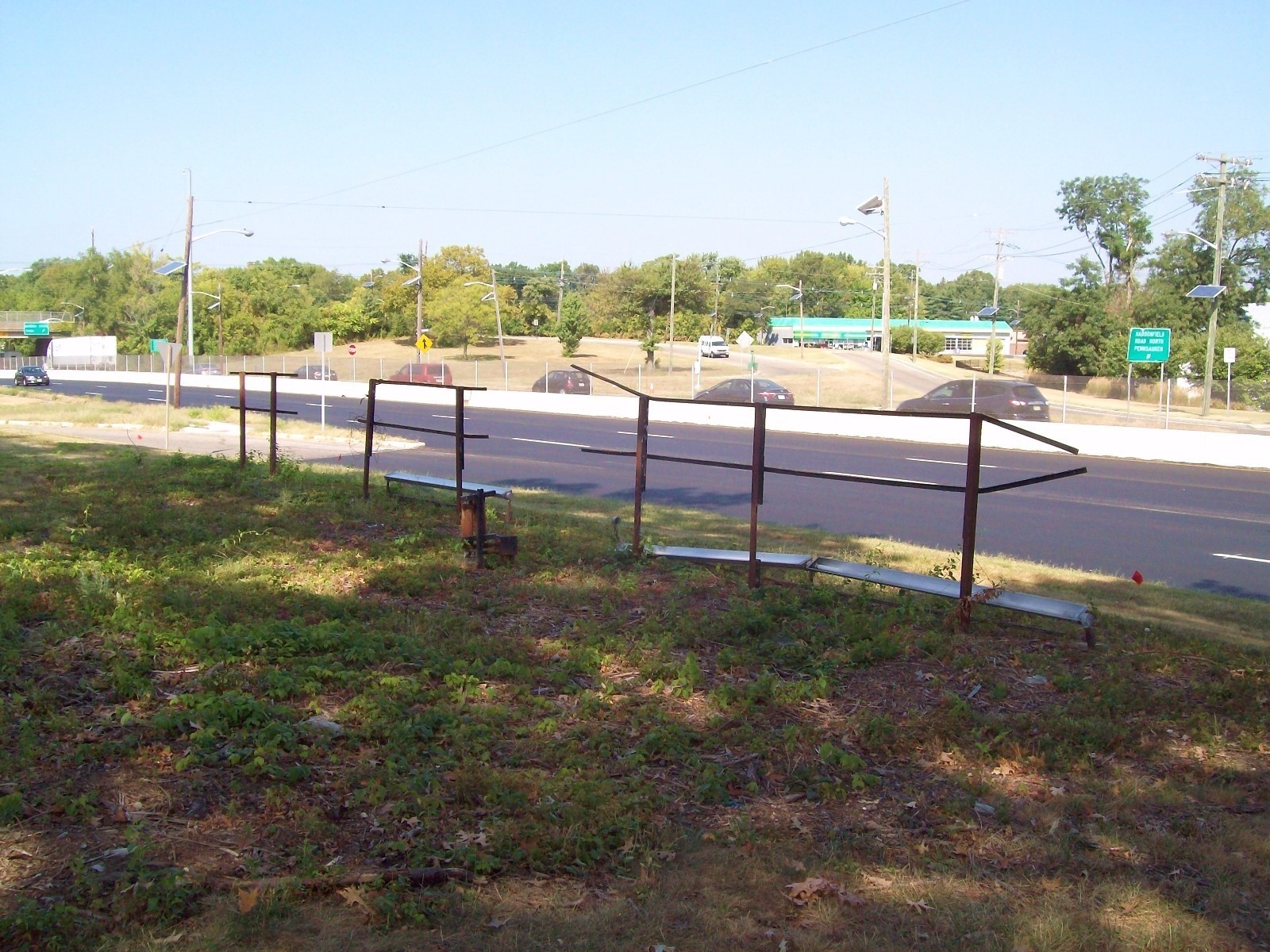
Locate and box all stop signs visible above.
[349,345,356,355]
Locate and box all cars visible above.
[188,363,222,375]
[893,378,1050,421]
[693,378,794,406]
[14,366,49,386]
[40,318,64,323]
[290,365,337,381]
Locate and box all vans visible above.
[698,335,729,358]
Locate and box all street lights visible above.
[839,216,890,411]
[186,228,254,372]
[464,282,505,359]
[382,258,421,341]
[776,285,804,359]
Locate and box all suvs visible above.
[532,370,590,395]
[389,364,453,385]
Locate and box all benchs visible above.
[806,554,1097,650]
[385,470,514,523]
[613,517,813,593]
